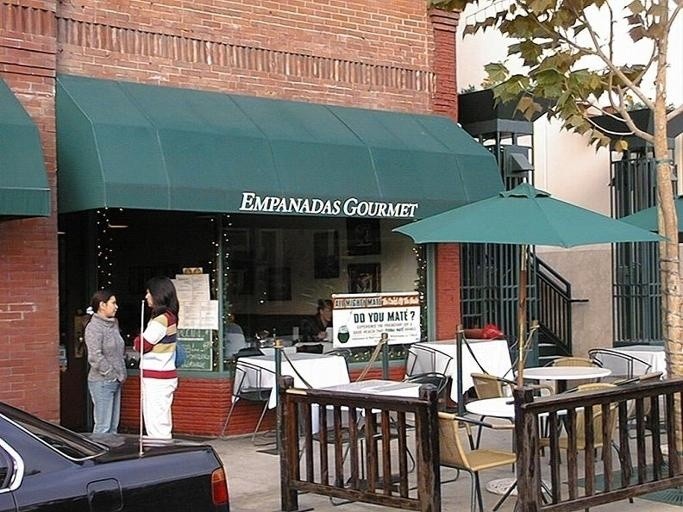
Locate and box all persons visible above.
[83,291,127,435]
[304,298,332,341]
[132,276,180,439]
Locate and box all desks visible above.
[596,340,666,381]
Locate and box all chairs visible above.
[219,333,652,510]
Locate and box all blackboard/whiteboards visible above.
[176,329,213,372]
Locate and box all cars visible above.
[1,400,230,512]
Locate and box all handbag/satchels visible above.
[176,345,186,368]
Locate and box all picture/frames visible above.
[312,217,381,292]
[225,225,291,302]
